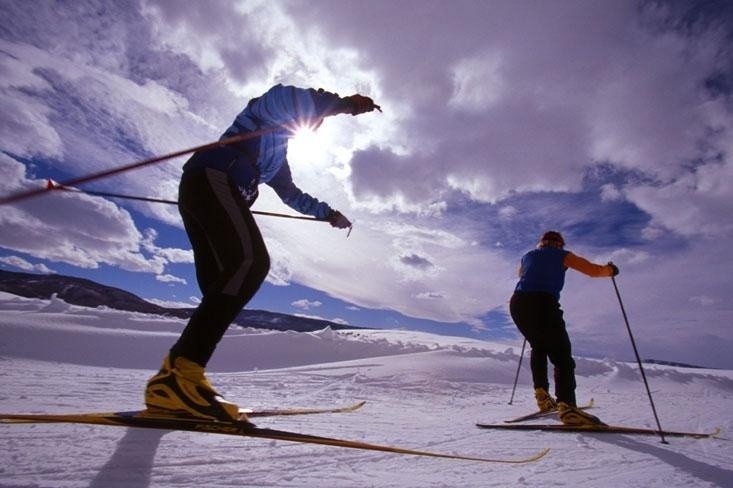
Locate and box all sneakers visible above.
[535,388,557,410]
[143,350,239,421]
[558,402,610,428]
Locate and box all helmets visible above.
[540,230,565,246]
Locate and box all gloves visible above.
[343,93,374,116]
[326,209,351,229]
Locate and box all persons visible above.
[508,230,621,429]
[143,80,375,424]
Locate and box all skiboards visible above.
[0,401,551,464]
[475,398,721,436]
